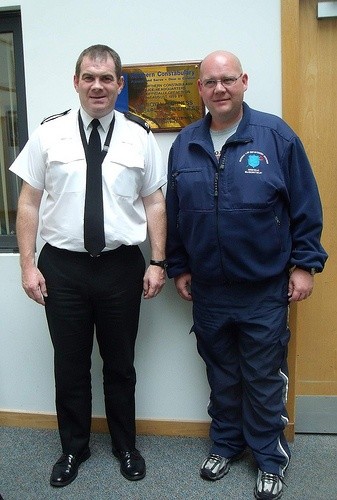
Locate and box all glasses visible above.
[199,73,244,89]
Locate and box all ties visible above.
[83,118,107,258]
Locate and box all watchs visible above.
[149,260,167,271]
[296,264,316,277]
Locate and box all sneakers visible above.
[253,466,288,500]
[199,452,232,481]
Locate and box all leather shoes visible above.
[112,445,147,481]
[49,448,91,487]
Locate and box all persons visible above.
[127,69,158,128]
[166,51,329,500]
[8,45,167,486]
